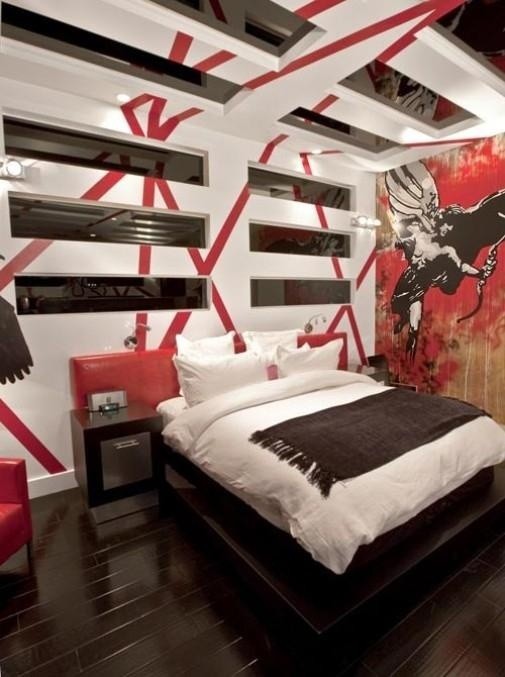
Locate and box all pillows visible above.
[173,327,349,404]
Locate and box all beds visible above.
[155,369,505,672]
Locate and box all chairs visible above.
[1,457,32,575]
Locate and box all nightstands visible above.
[70,399,162,524]
[350,364,391,385]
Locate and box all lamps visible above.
[123,320,148,350]
[303,312,327,332]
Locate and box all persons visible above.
[392,203,493,366]
